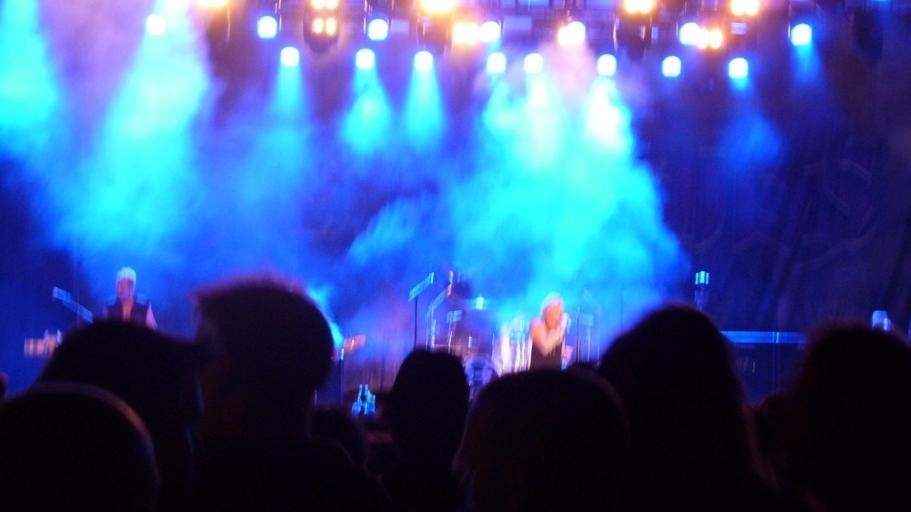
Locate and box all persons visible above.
[1,267,910,510]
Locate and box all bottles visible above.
[352,384,377,416]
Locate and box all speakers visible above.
[721,331,810,400]
